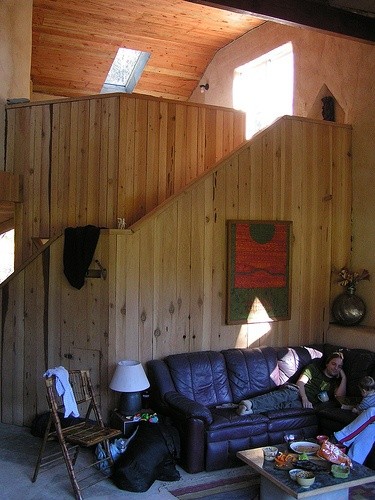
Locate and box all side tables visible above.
[111,407,160,439]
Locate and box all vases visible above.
[333,285,366,326]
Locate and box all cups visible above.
[263,446,278,461]
[317,391,329,403]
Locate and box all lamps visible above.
[200,84,210,93]
[109,360,150,414]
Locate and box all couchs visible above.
[146,344,375,474]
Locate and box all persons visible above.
[352,376,375,417]
[236,351,347,416]
[329,406,375,465]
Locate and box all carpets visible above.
[157,474,375,500]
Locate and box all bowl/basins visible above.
[334,444,346,453]
[316,435,329,444]
[284,433,304,444]
[296,471,316,488]
[289,468,304,481]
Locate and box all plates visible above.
[273,462,292,470]
[290,441,321,455]
[293,459,338,474]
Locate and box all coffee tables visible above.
[237,438,375,500]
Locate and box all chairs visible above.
[30,368,121,500]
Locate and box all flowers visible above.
[334,266,370,288]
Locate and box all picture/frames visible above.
[226,219,293,326]
